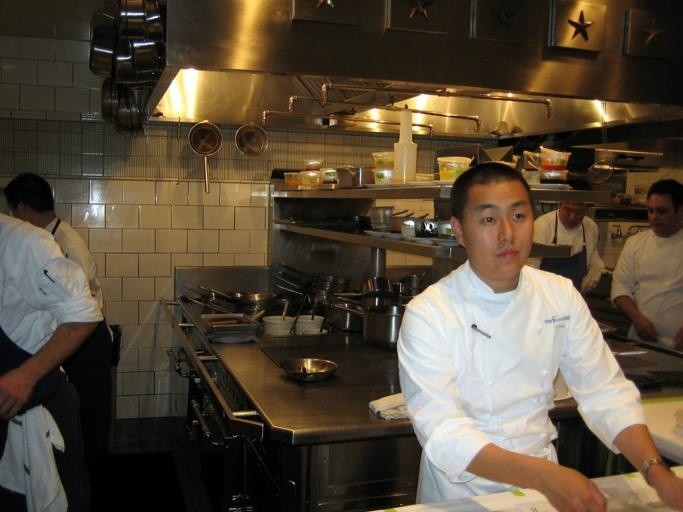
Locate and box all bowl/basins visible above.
[370,206,457,239]
[262,314,329,336]
[371,150,395,184]
[277,358,338,381]
[235,122,269,156]
[283,169,338,188]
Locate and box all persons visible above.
[520,195,602,301]
[0,170,109,509]
[606,175,682,353]
[396,158,682,511]
[0,211,104,511]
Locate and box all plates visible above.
[365,230,460,247]
[363,182,434,188]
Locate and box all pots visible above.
[187,120,223,193]
[336,164,376,188]
[199,285,276,305]
[89,0,166,135]
[276,262,428,346]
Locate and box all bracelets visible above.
[639,455,664,479]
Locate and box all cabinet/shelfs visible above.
[266,186,611,290]
